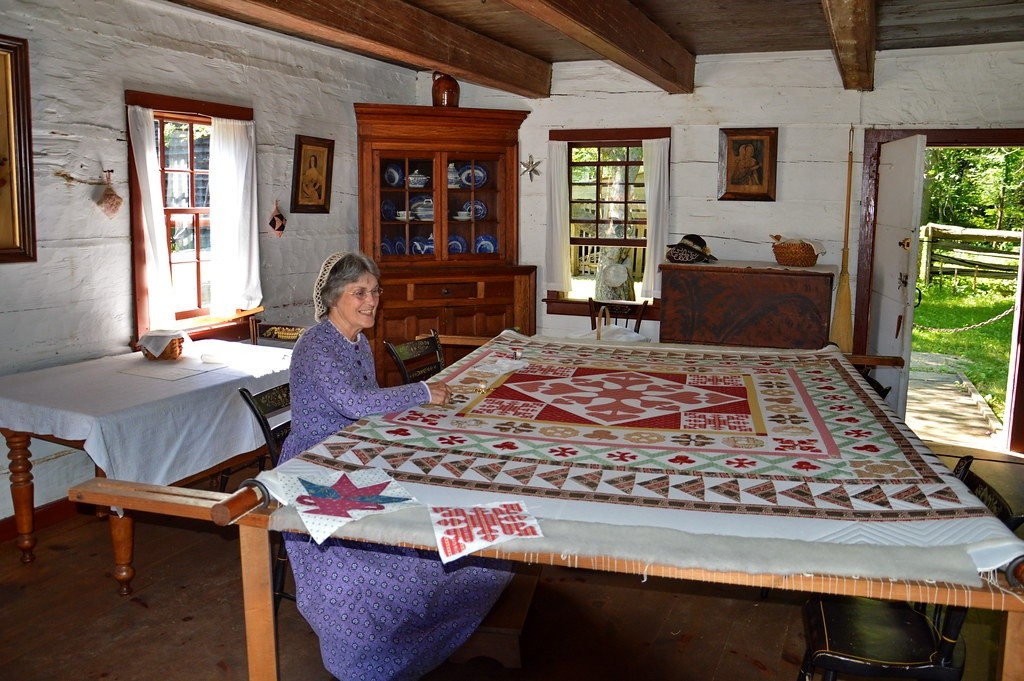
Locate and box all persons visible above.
[276,250,515,681]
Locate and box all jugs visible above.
[414,233,435,254]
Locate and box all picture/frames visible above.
[0,35,37,263]
[717,126,778,202]
[290,134,335,214]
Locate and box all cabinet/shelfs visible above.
[353,103,537,389]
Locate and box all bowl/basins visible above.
[385,164,403,187]
[412,199,434,221]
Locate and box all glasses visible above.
[341,286,384,299]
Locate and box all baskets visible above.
[140,332,186,361]
[772,241,820,268]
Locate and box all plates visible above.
[452,216,471,221]
[395,217,415,221]
[381,199,396,221]
[458,165,488,188]
[448,234,468,253]
[462,200,487,221]
[476,233,498,253]
[381,236,406,254]
[410,237,428,254]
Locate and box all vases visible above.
[432,70,460,107]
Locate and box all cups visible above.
[457,212,472,217]
[396,210,411,217]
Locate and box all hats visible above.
[666,234,719,262]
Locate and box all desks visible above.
[657,260,838,350]
[0,338,295,596]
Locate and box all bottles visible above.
[433,71,460,107]
[404,170,430,187]
[447,163,461,188]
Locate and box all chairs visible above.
[797,455,1024,681]
[383,329,445,384]
[237,384,307,616]
[589,298,648,334]
